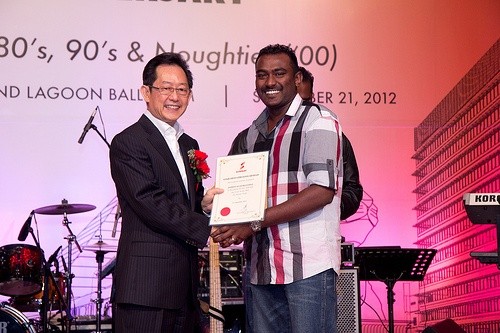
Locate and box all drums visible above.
[0,243,46,297]
[21,272,68,311]
[0,301,46,333]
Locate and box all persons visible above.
[297,66,364,220]
[109,51,224,333]
[210,44,344,333]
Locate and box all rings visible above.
[231,237,237,242]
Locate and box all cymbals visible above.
[81,249,117,253]
[82,244,119,249]
[32,203,97,216]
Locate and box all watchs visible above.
[250,220,263,236]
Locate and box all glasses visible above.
[149,85,190,95]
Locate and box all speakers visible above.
[336,267,362,333]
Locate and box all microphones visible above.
[112,205,120,236]
[78,108,97,143]
[47,245,62,264]
[18,210,35,241]
